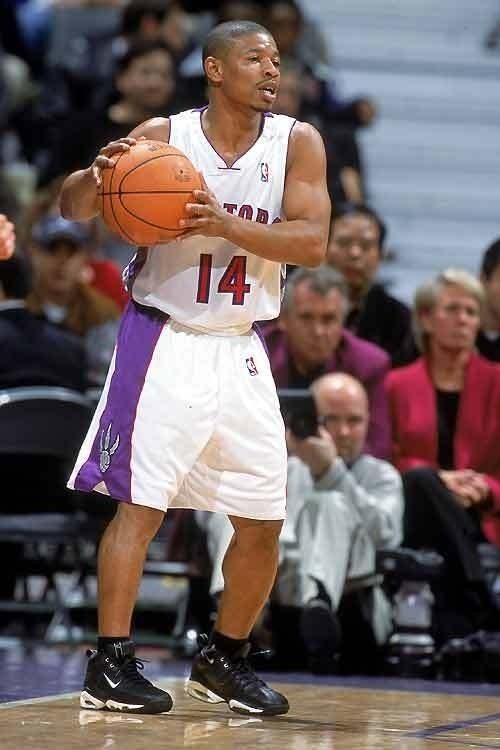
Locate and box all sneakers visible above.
[80,638,174,718]
[184,632,290,718]
[174,627,273,664]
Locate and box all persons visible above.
[254,264,394,465]
[380,265,500,630]
[0,0,416,367]
[475,239,499,368]
[201,371,404,649]
[57,20,332,715]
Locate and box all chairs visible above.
[88,390,200,636]
[0,387,97,636]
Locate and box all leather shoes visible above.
[302,597,340,677]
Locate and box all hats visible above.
[29,214,88,250]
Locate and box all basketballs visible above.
[96,141,202,245]
